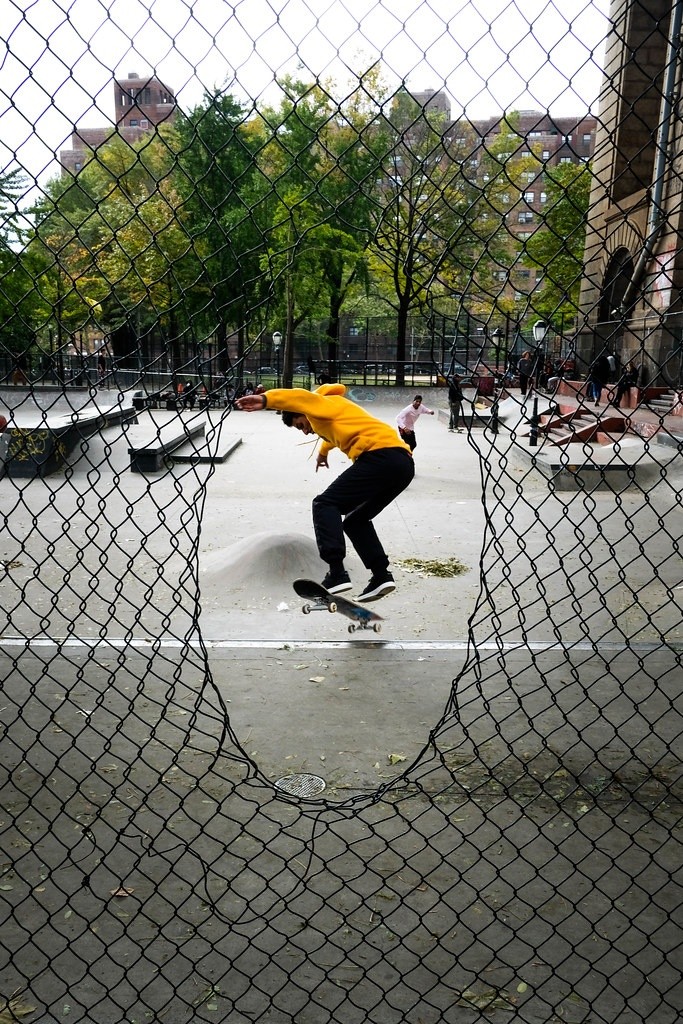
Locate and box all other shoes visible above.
[448,429,455,433]
[615,403,621,407]
[454,428,462,431]
[584,397,589,401]
[595,403,599,406]
[586,398,595,402]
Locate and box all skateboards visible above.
[448,428,464,434]
[293,579,384,633]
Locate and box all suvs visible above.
[361,364,396,375]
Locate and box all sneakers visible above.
[321,571,353,594]
[357,573,397,603]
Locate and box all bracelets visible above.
[262,396,266,410]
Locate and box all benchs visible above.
[158,389,220,409]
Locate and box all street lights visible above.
[533,320,545,389]
[272,331,283,414]
[491,325,505,435]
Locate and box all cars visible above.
[441,363,466,375]
[340,364,364,375]
[258,367,277,374]
[294,366,313,375]
[403,364,431,375]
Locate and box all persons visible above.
[396,395,433,453]
[316,368,330,385]
[98,349,105,390]
[13,351,27,385]
[609,361,639,408]
[182,379,198,412]
[233,381,415,603]
[584,353,615,407]
[224,382,267,409]
[517,350,564,397]
[447,373,463,430]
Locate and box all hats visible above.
[257,384,263,388]
